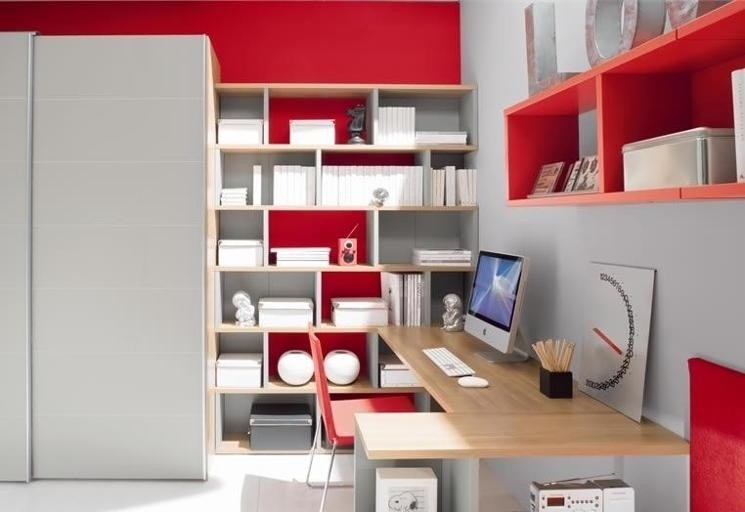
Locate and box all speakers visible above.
[338,238,357,265]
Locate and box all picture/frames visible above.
[530,161,565,194]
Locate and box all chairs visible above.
[307,322,417,512]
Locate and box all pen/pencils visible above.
[532,336,575,373]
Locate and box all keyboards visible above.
[422,347,476,377]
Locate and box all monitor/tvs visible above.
[463,249,531,363]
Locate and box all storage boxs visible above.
[217,239,264,268]
[330,298,389,328]
[217,119,263,144]
[622,127,734,190]
[248,404,311,450]
[258,297,313,326]
[289,120,335,144]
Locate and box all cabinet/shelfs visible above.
[213,80,478,455]
[1,32,222,482]
[504,0,745,207]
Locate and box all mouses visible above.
[458,376,489,388]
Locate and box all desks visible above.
[353,326,690,512]
